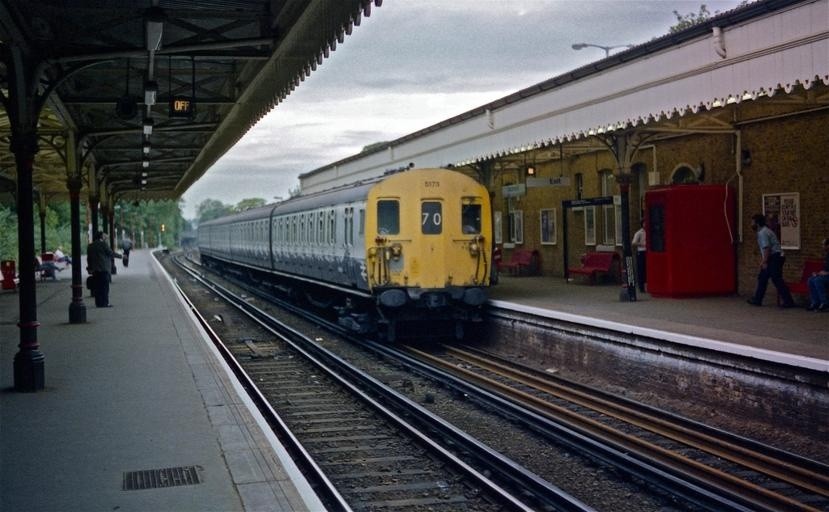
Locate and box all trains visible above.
[196,167,498,347]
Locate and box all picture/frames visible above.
[514,209,524,244]
[761,192,803,250]
[539,207,558,246]
[583,205,597,246]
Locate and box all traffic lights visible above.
[161,224,166,233]
[169,97,194,116]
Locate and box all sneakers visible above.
[746,296,763,306]
[806,303,828,313]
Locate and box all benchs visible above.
[566,252,620,284]
[784,258,826,308]
[497,248,541,276]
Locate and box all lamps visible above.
[141,21,164,185]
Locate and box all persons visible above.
[122,236,132,267]
[747,215,796,309]
[632,219,646,293]
[35,254,64,281]
[55,245,72,266]
[86,231,127,307]
